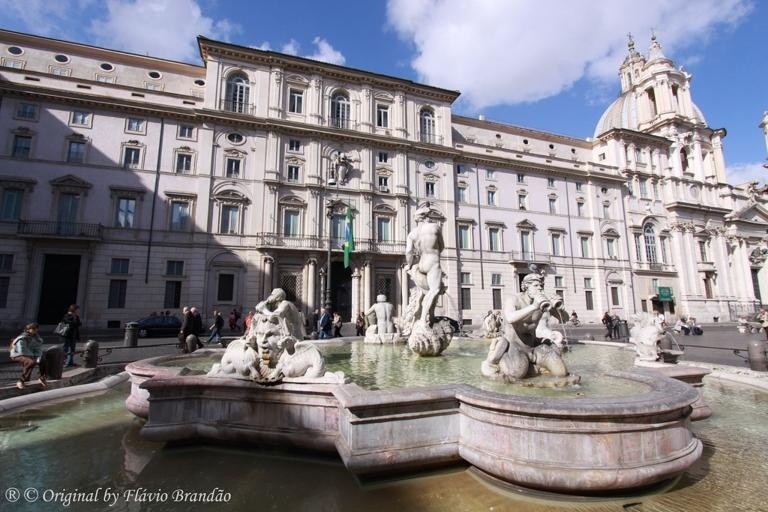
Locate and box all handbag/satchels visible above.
[53,319,70,338]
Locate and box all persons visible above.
[404,208,444,324]
[9,322,44,388]
[570,309,578,324]
[307,306,368,339]
[178,305,253,354]
[756,308,768,341]
[601,312,613,339]
[62,305,81,366]
[364,294,395,334]
[674,317,690,335]
[495,311,502,324]
[255,288,305,342]
[610,311,620,339]
[655,311,664,326]
[495,273,569,377]
[738,315,752,334]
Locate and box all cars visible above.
[126,316,181,339]
[437,315,462,334]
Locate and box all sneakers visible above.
[15,380,27,390]
[37,376,49,389]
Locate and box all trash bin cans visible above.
[124,321,139,347]
[619,320,630,338]
[713,318,718,323]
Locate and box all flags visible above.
[343,200,354,267]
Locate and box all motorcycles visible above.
[679,318,704,337]
